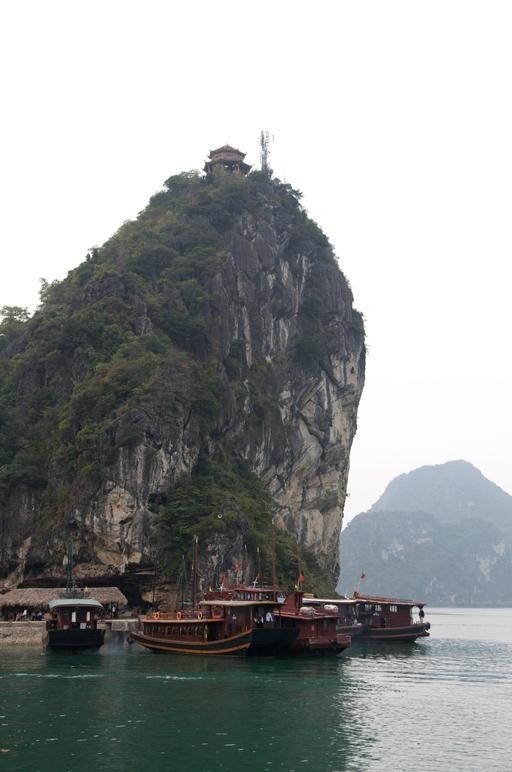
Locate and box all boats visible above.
[41,596,106,652]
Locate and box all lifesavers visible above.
[197,612,202,620]
[176,612,181,619]
[154,612,160,619]
[212,607,224,618]
